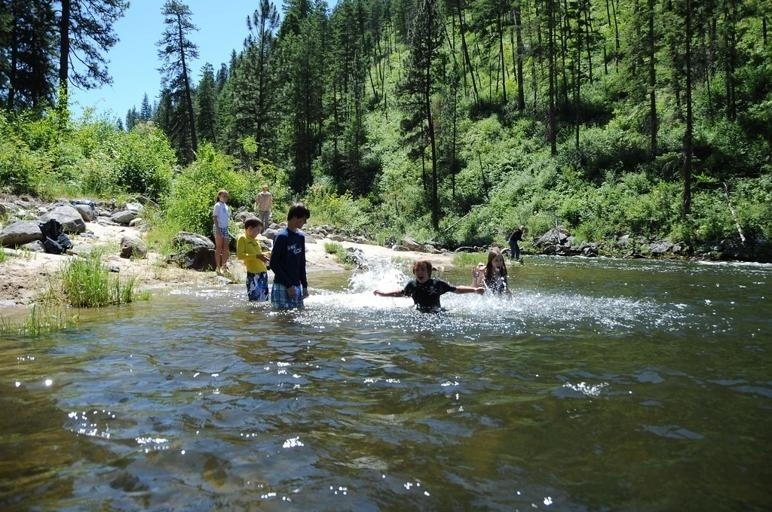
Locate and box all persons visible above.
[211,189,231,275]
[267,203,311,310]
[237,218,271,302]
[507,225,526,260]
[473,247,510,299]
[373,257,487,317]
[253,184,273,231]
